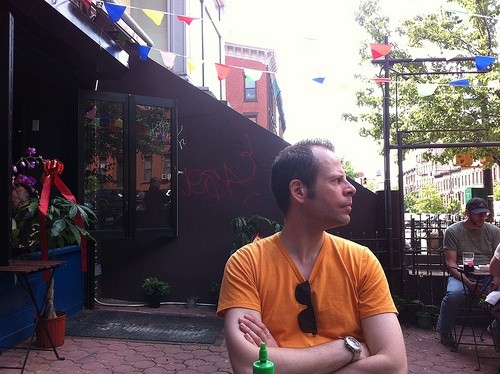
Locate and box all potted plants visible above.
[10,196,98,348]
[391,295,439,330]
[141,277,169,308]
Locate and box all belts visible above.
[468,275,490,280]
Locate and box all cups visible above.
[462,252,475,272]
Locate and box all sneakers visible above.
[487,323,495,341]
[436,327,456,347]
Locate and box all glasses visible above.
[295,279,317,335]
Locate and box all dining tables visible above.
[450,265,500,370]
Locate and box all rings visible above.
[266,332,269,334]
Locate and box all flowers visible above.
[11,148,45,199]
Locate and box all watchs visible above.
[344,336,362,362]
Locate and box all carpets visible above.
[64,309,225,344]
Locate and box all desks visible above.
[0,261,70,374]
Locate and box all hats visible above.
[466,197,491,214]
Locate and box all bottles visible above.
[253,342,274,374]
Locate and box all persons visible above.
[12,186,31,239]
[437,198,500,346]
[487,242,500,340]
[145,178,170,208]
[216,138,407,374]
